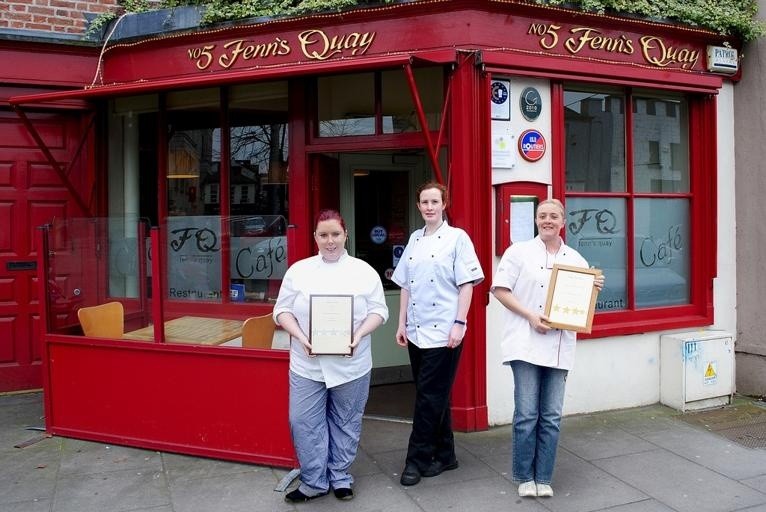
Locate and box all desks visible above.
[122,313,245,346]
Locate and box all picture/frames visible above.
[540,264,604,335]
[309,294,356,357]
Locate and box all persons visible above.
[272,209,389,502]
[492,198,605,498]
[391,182,484,488]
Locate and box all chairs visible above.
[77,300,124,342]
[240,312,277,350]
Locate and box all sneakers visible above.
[518,480,553,497]
[284,488,330,503]
[334,488,353,501]
[401,458,458,485]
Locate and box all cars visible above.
[243,215,267,236]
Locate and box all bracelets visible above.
[454,320,466,325]
